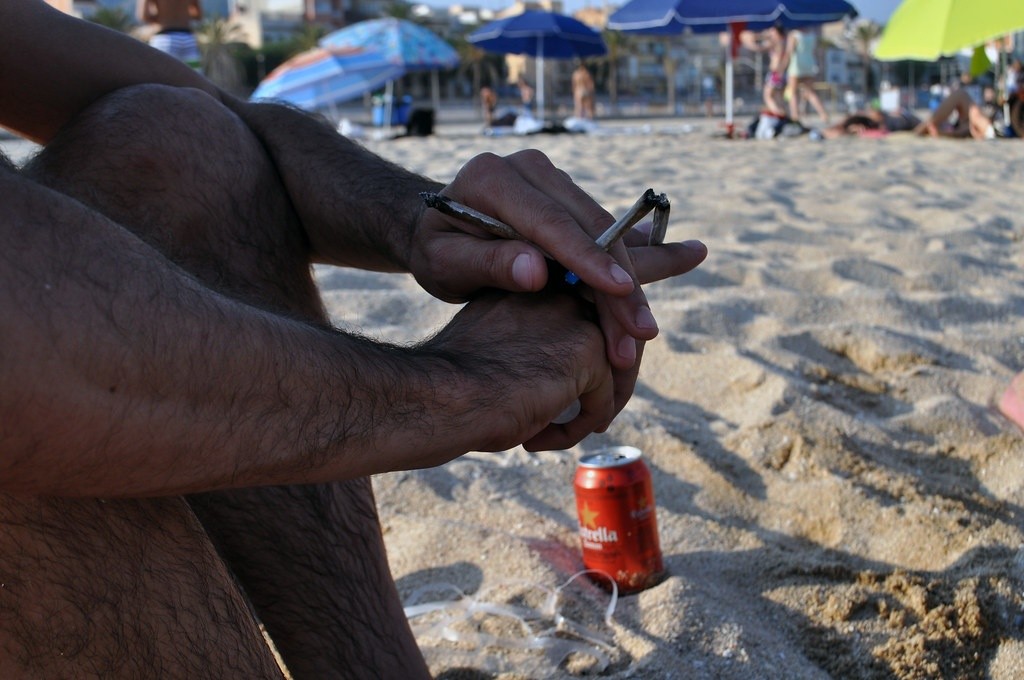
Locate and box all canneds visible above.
[574,443,664,596]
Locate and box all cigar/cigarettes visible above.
[564,189,658,286]
[651,193,670,246]
[419,191,553,259]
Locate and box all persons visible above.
[829,57,1023,138]
[0,0,707,680]
[136,0,205,78]
[516,76,535,114]
[479,85,496,126]
[742,23,831,126]
[569,64,594,119]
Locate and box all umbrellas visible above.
[873,0,1024,62]
[607,0,858,137]
[464,12,606,120]
[247,13,460,133]
